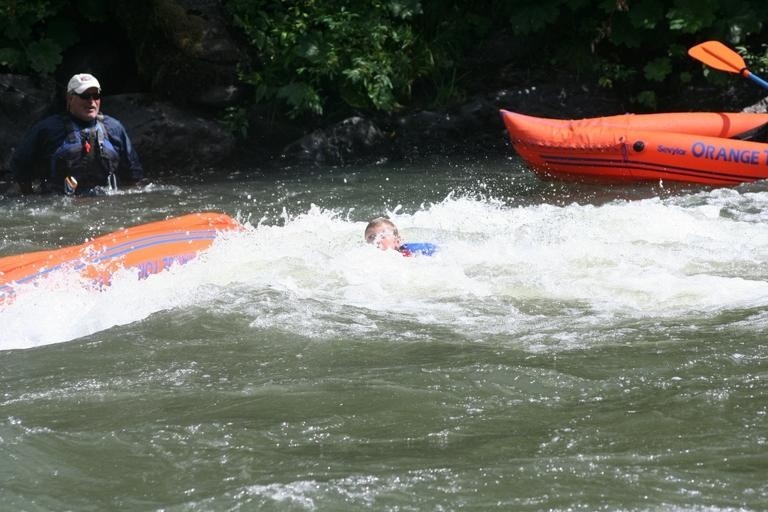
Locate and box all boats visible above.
[1,211,246,309]
[499,105,768,190]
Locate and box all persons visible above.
[11,71,144,194]
[363,217,436,257]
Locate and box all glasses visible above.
[71,92,101,99]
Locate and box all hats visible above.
[67,73,100,95]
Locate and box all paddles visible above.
[687,40,768,89]
